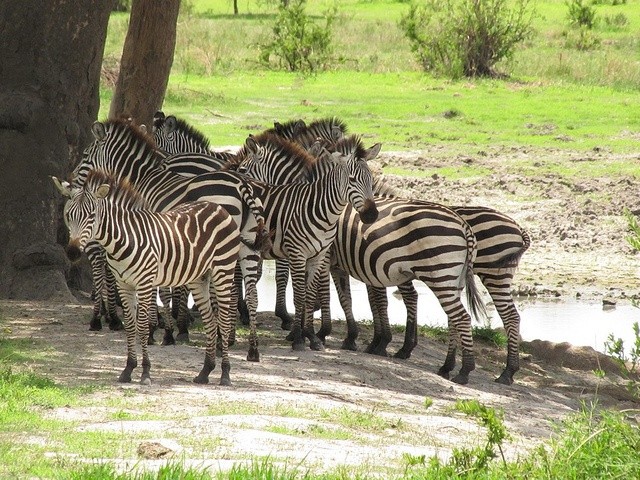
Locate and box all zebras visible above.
[48,112,529,388]
